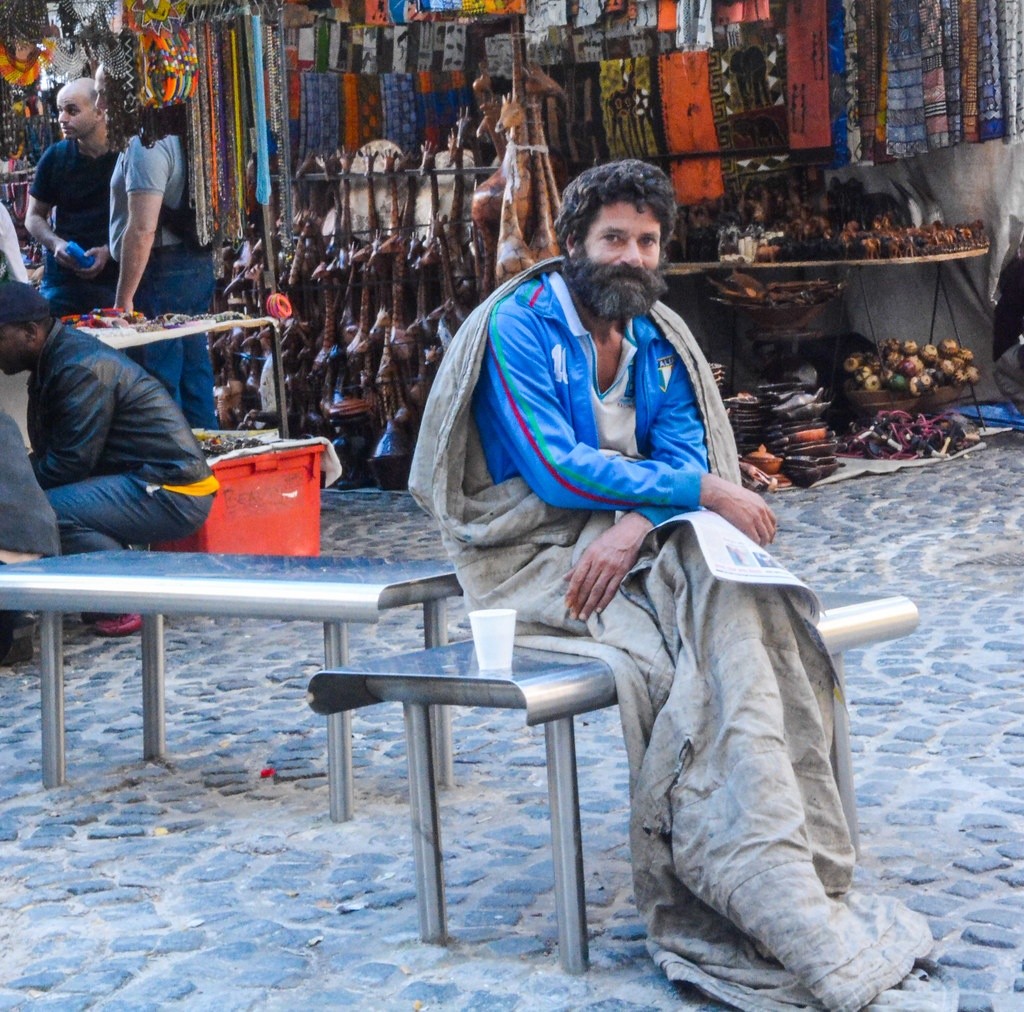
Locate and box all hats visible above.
[0,280,49,322]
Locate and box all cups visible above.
[468,609,519,672]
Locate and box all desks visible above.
[669,248,990,445]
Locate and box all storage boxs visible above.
[148,432,327,556]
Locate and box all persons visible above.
[409,158,956,1012]
[0,63,218,662]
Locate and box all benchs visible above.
[309,592,920,975]
[0,548,464,823]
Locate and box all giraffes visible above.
[272,60,567,434]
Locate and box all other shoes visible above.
[79,611,142,636]
[0,612,35,666]
